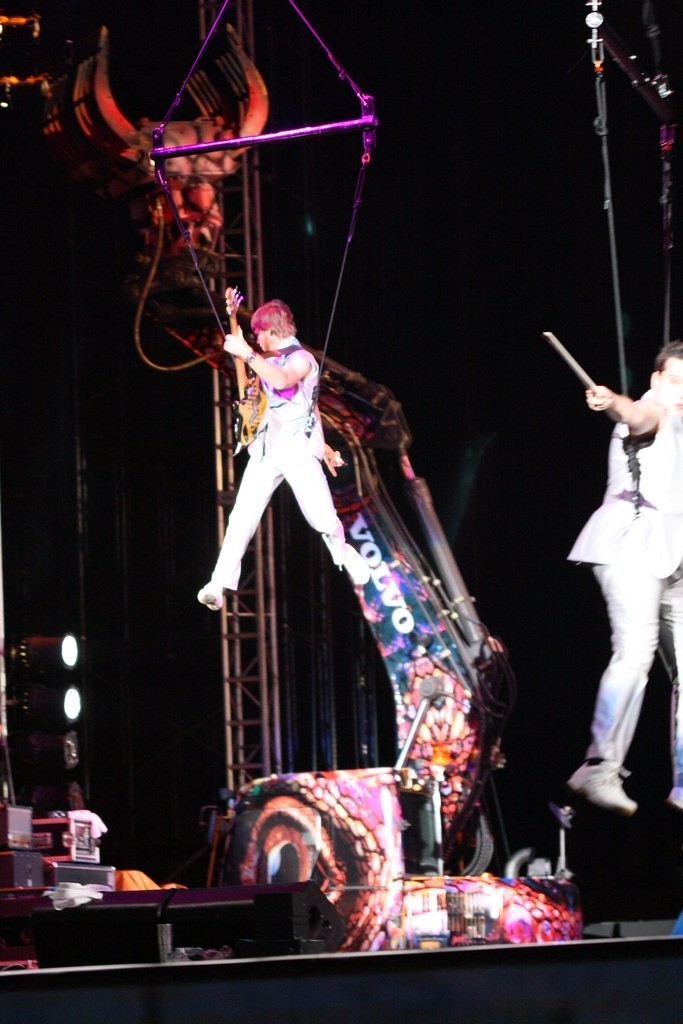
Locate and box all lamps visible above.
[8,780,84,818]
[6,731,79,770]
[7,685,81,724]
[7,633,79,671]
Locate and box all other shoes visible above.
[199,583,224,611]
[339,544,370,584]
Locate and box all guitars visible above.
[224,285,268,457]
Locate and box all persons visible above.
[564,344,683,810]
[195,300,370,610]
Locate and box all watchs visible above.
[243,351,257,363]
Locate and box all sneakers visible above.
[567,759,638,817]
[667,787,683,811]
